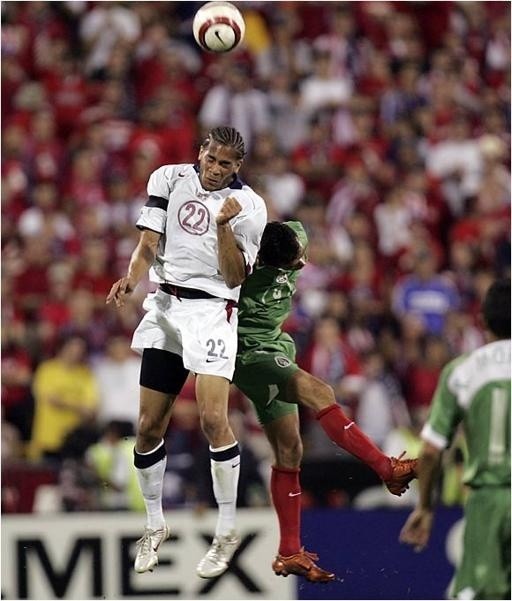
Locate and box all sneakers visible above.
[272,545,336,584]
[383,450,420,498]
[195,526,242,579]
[134,524,171,574]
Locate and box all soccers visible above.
[192,2,245,52]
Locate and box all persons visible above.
[3,1,512,516]
[231,217,421,583]
[104,124,269,580]
[395,273,511,598]
